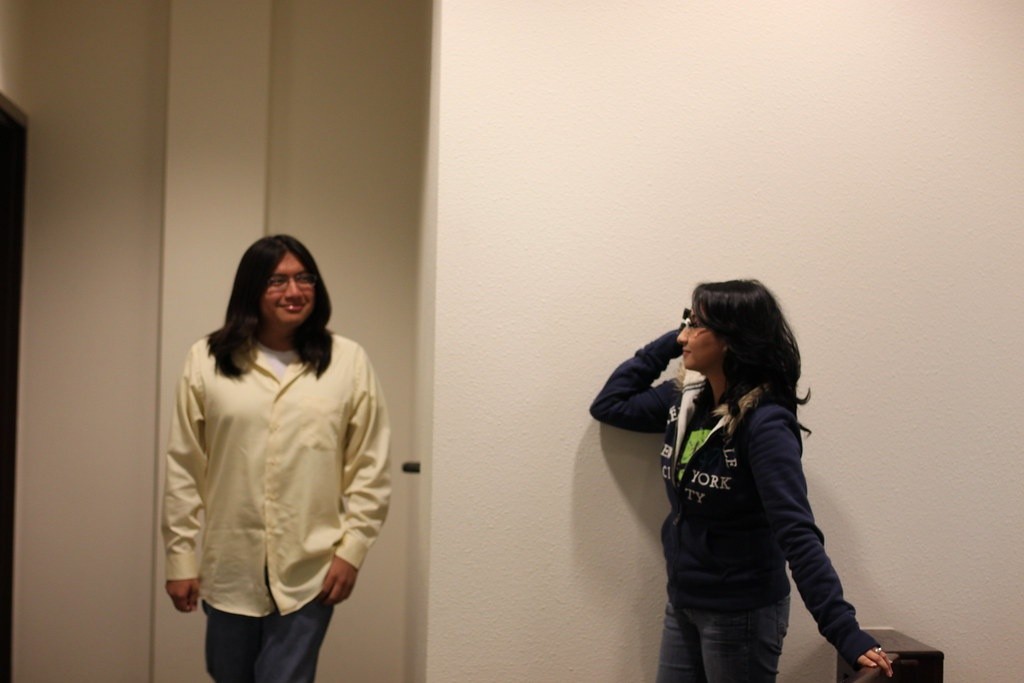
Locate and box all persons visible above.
[160,234,392,683]
[587,277,893,683]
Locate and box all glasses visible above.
[685,317,714,337]
[267,271,315,292]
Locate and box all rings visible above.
[875,647,881,653]
